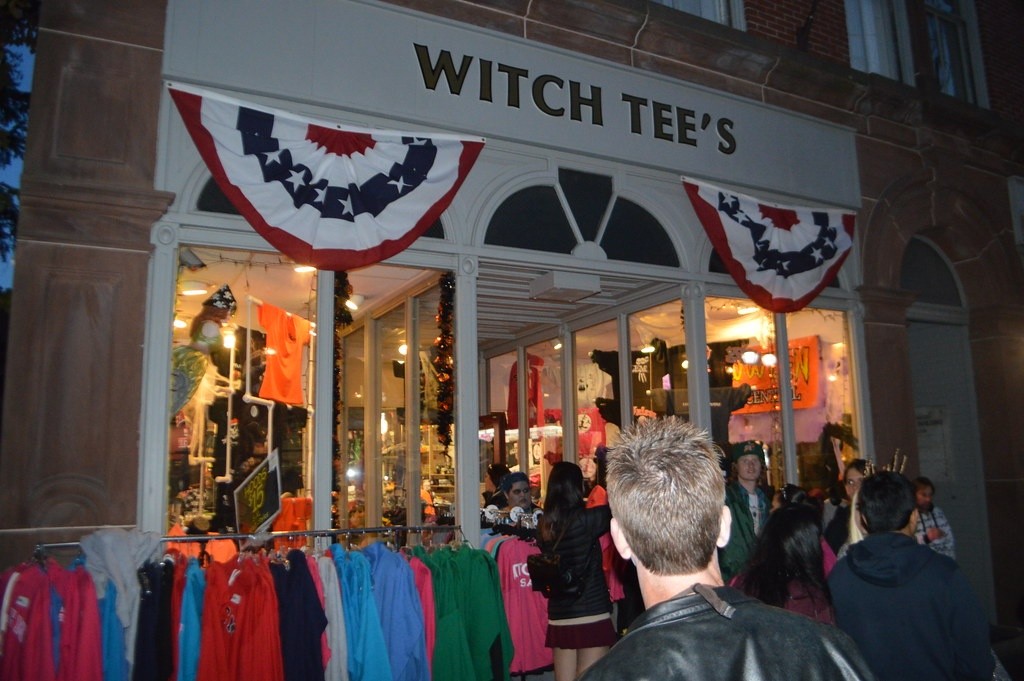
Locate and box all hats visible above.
[732,440,768,472]
[487,464,512,488]
[501,472,528,491]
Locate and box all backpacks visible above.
[527,509,597,602]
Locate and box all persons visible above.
[717,440,772,586]
[382,495,407,545]
[912,476,957,561]
[828,470,997,681]
[500,471,544,518]
[824,458,880,558]
[727,502,837,628]
[573,414,878,681]
[769,483,836,583]
[838,488,869,550]
[535,462,614,681]
[348,499,366,529]
[420,488,436,548]
[482,463,512,510]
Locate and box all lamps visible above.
[391,351,405,364]
[222,323,239,348]
[178,279,215,296]
[174,316,188,329]
[345,295,365,310]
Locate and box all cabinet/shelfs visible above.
[399,423,455,498]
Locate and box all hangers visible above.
[26,507,543,595]
